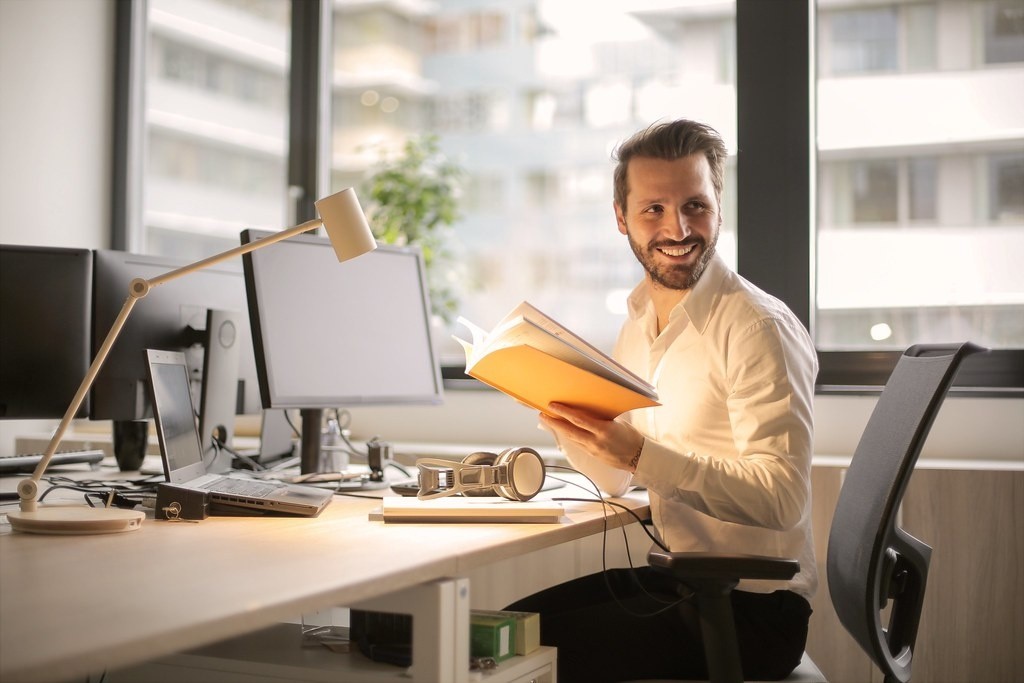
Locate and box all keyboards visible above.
[0,448,105,474]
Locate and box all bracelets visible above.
[629,437,645,472]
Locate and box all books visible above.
[450,300,663,421]
[365,495,566,524]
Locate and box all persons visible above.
[500,118,817,683]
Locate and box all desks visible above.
[0,464,654,683]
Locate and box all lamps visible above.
[6,188,378,536]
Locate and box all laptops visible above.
[142,349,335,516]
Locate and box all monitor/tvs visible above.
[0,230,447,493]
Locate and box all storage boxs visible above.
[470,609,540,656]
[349,608,515,663]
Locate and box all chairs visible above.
[646,341,992,682]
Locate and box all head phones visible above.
[416,447,545,501]
